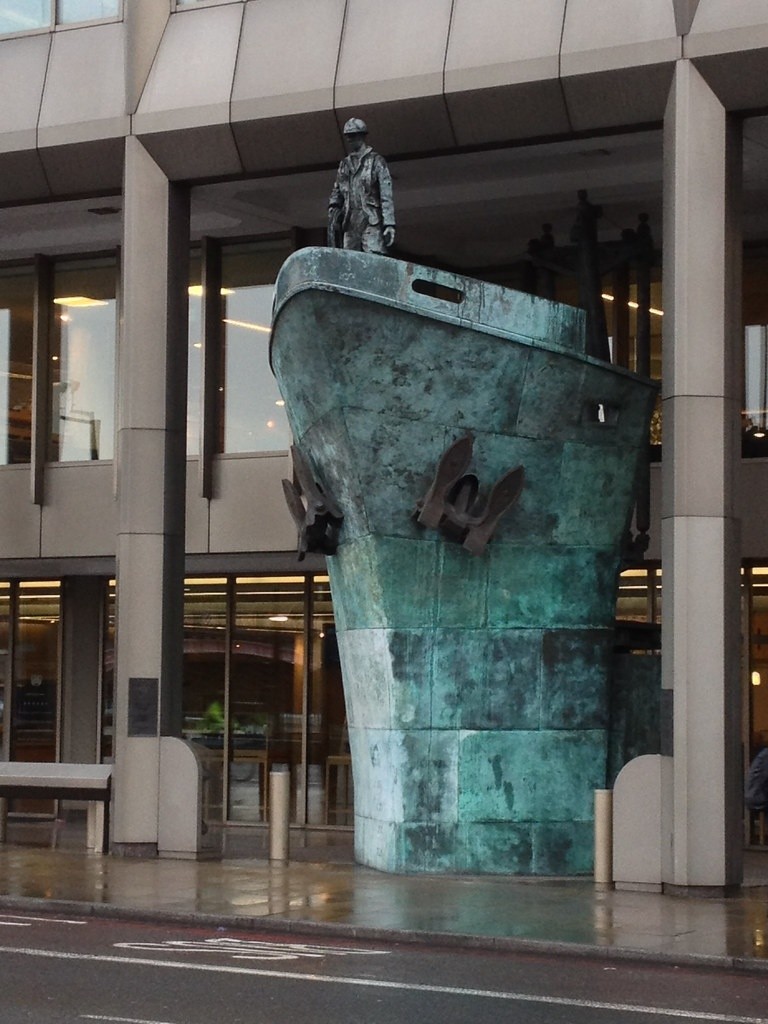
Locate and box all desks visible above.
[194,736,326,824]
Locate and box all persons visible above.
[541,223,555,245]
[527,238,549,266]
[617,213,653,259]
[328,118,395,258]
[574,189,603,235]
